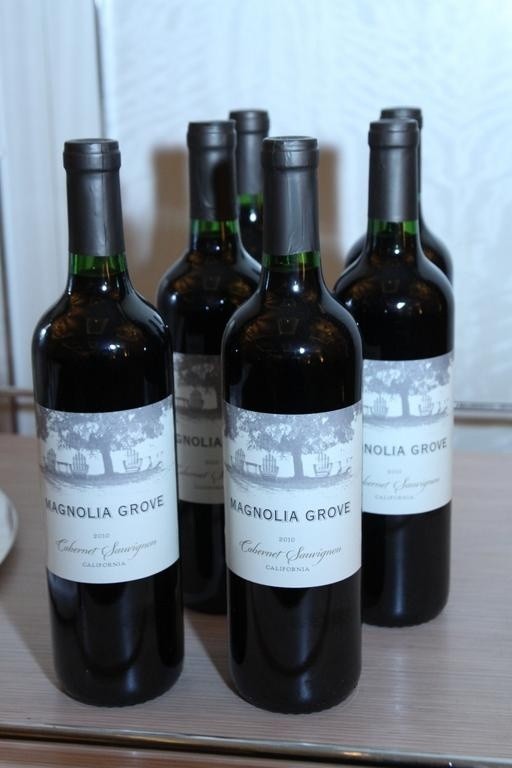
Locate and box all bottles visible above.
[29,136,187,712]
[155,117,263,623]
[217,132,365,717]
[329,114,457,631]
[220,107,273,268]
[342,104,454,282]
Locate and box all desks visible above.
[0,387,512,768]
